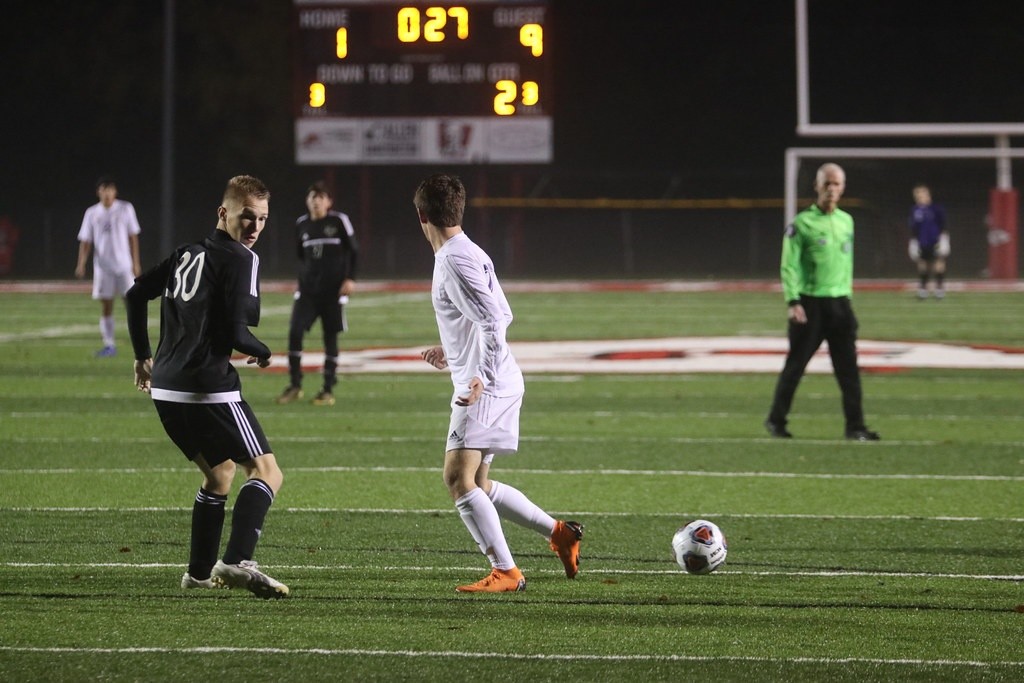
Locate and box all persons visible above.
[126,174,289,602]
[908,182,951,300]
[414,172,584,593]
[764,162,883,442]
[74,174,141,357]
[274,180,359,407]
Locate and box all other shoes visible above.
[275,386,305,403]
[846,427,881,441]
[765,420,791,439]
[95,346,114,356]
[311,390,335,404]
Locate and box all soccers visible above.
[671,519,726,575]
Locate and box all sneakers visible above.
[455,568,527,593]
[180,572,229,591]
[549,520,584,580]
[210,560,289,601]
